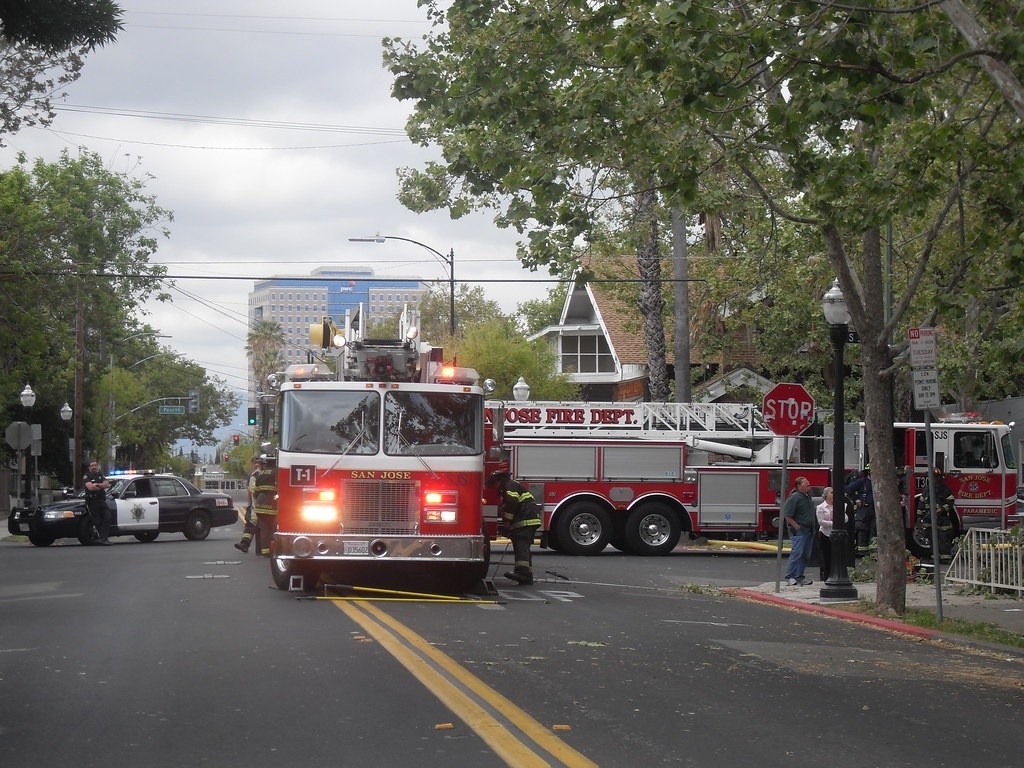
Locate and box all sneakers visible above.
[797,578,812,585]
[785,578,797,585]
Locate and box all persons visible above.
[494,470,542,586]
[235,454,278,558]
[845,462,912,547]
[81,459,115,546]
[916,468,956,564]
[815,488,847,581]
[783,476,817,585]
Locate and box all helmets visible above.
[266,454,277,460]
[931,467,944,475]
[257,453,267,462]
[861,462,870,472]
[490,470,513,486]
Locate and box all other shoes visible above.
[102,538,113,545]
[504,571,533,585]
[235,542,248,552]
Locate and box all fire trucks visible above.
[482,398,834,557]
[259,301,507,595]
[836,411,1020,560]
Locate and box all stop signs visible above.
[762,382,816,438]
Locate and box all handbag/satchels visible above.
[854,506,869,530]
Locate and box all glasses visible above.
[801,481,809,485]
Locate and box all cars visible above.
[8,469,238,547]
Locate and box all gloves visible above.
[856,499,862,507]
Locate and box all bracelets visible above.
[96,484,99,487]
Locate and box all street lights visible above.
[20,381,37,509]
[108,332,173,464]
[349,233,457,368]
[819,277,860,603]
[59,402,74,487]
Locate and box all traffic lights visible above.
[248,408,256,425]
[225,455,229,459]
[310,316,332,349]
[234,435,239,446]
